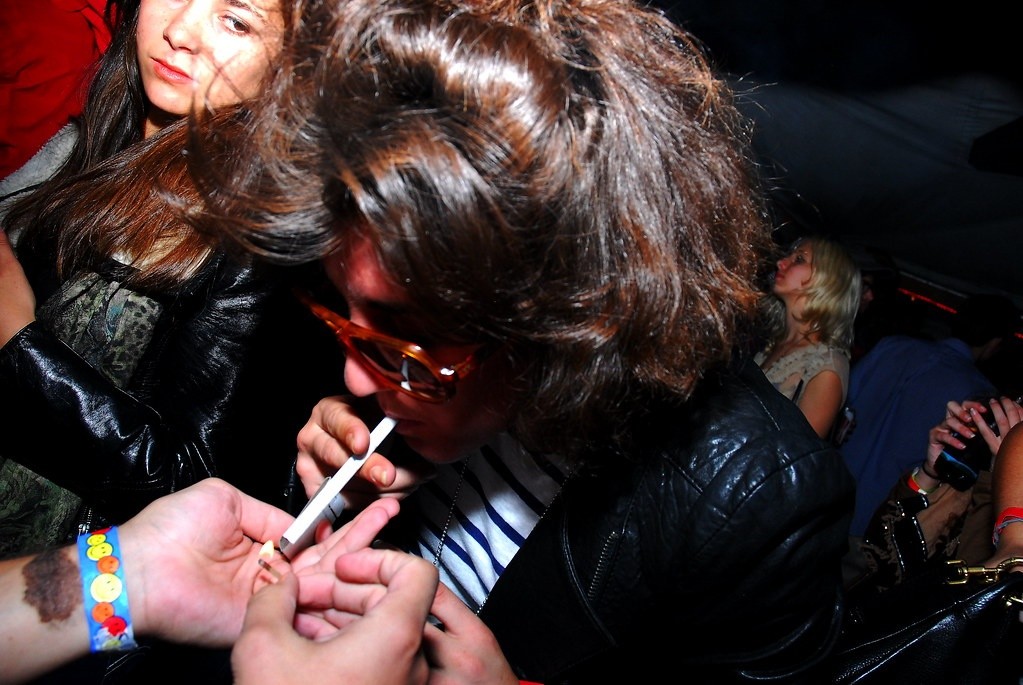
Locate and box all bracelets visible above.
[909,468,943,495]
[77,525,138,652]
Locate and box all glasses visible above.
[288,287,501,403]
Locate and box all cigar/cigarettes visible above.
[282,416,398,544]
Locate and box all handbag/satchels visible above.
[821,558,1023,685]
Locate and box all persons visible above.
[754,232,863,442]
[836,398,1023,685]
[845,292,1015,546]
[0,0,840,685]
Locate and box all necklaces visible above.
[88,277,136,343]
[762,332,809,375]
[432,451,579,615]
